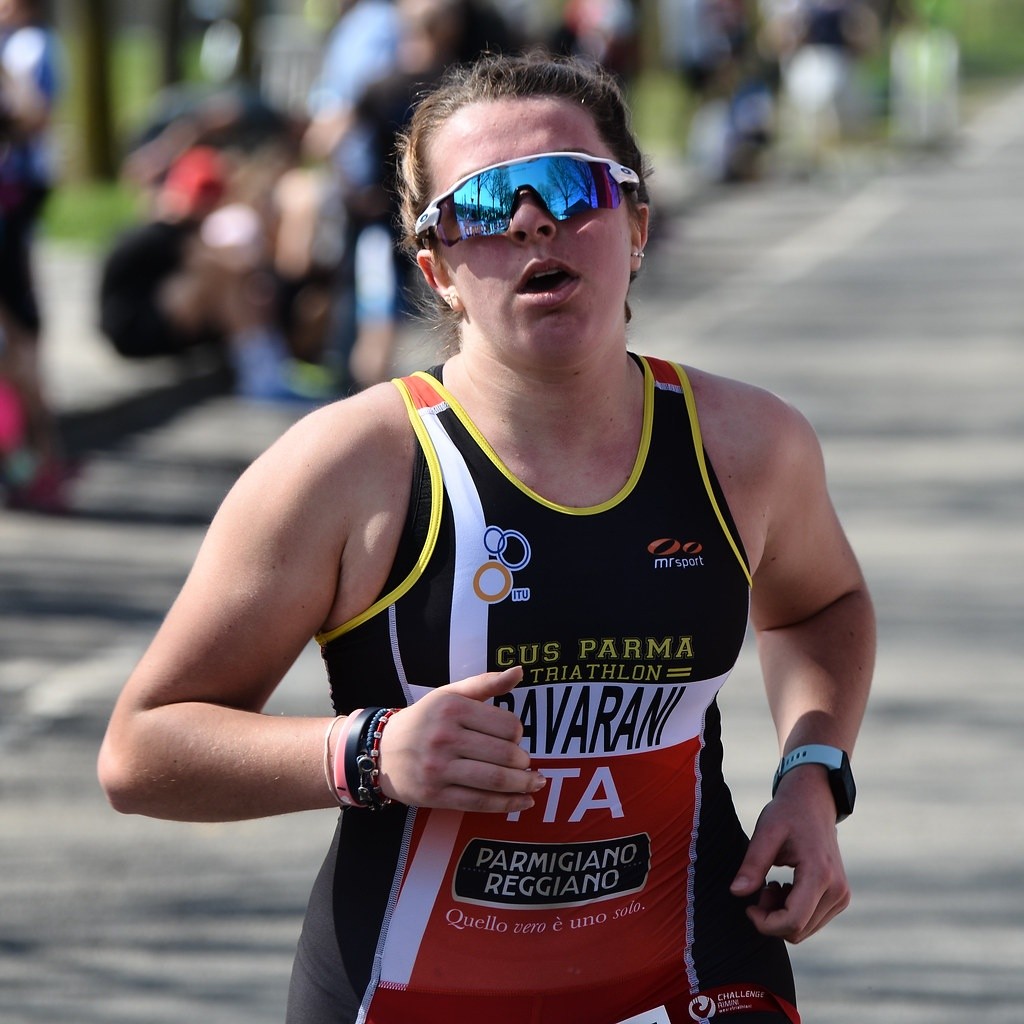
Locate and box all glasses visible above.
[415,152,640,252]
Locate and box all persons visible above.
[99,51,872,1024]
[0,0,63,433]
[463,208,506,236]
[99,0,457,403]
[555,0,880,184]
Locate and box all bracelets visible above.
[324,707,400,810]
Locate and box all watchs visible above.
[772,744,857,824]
